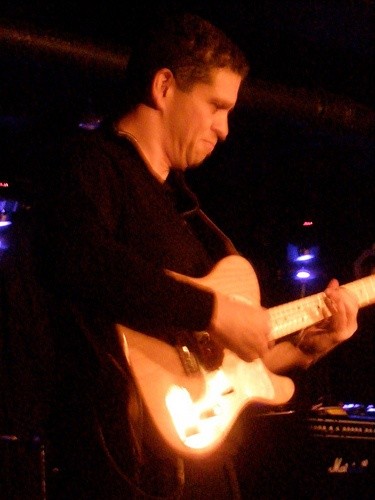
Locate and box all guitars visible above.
[115,254,375,462]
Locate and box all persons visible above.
[29,17,359,500]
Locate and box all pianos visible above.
[307,416,375,441]
